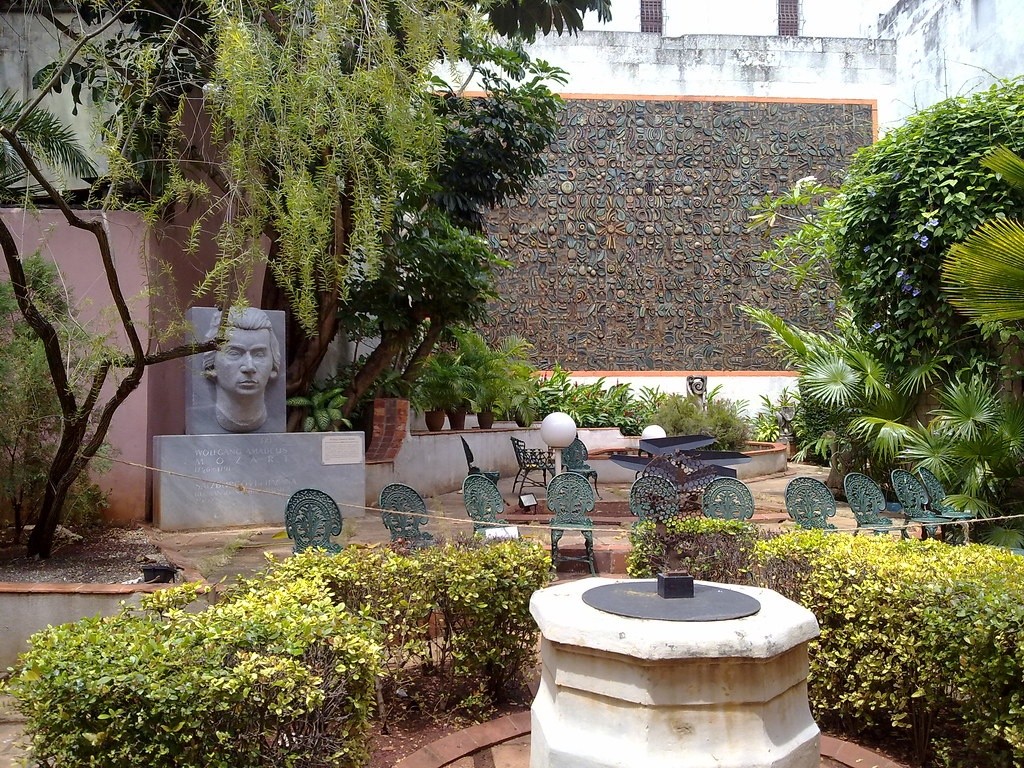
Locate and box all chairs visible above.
[702,477,754,523]
[893,469,956,546]
[785,476,835,529]
[511,436,558,497]
[286,488,344,555]
[379,483,435,544]
[544,472,595,535]
[560,438,604,499]
[843,472,892,537]
[462,473,510,533]
[919,466,977,545]
[629,475,678,525]
[460,434,510,507]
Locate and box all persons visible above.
[203,306,281,432]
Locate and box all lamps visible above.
[541,412,576,449]
[141,565,178,584]
